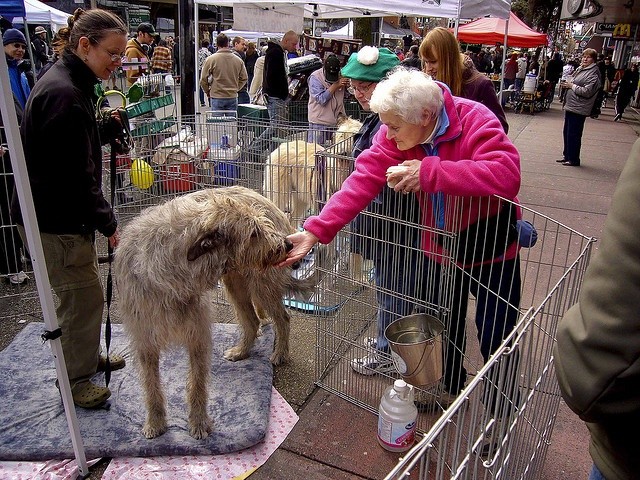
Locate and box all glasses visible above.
[12,42,27,49]
[346,83,373,93]
[87,37,127,62]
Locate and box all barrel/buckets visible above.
[195,161,216,185]
[384,312,447,387]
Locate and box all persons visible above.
[150,40,171,96]
[413,27,509,314]
[340,46,422,377]
[165,35,176,76]
[245,42,258,91]
[198,40,211,108]
[106,68,122,93]
[2,27,32,286]
[401,45,423,69]
[244,40,248,53]
[403,34,412,51]
[249,46,268,103]
[491,41,638,166]
[174,36,179,82]
[231,36,247,104]
[271,65,521,454]
[243,30,298,162]
[199,33,248,117]
[32,26,50,72]
[24,9,128,410]
[553,138,639,479]
[393,43,404,61]
[124,22,160,90]
[307,53,350,147]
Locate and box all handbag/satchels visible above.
[438,201,517,264]
[603,69,611,92]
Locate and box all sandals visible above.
[59,384,111,408]
[96,355,126,372]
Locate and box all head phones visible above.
[134,39,151,54]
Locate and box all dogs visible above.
[111,185,300,439]
[263,115,365,261]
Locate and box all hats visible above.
[2,28,27,45]
[138,22,160,36]
[324,54,341,80]
[340,46,401,82]
[34,26,47,34]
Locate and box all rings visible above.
[403,188,409,194]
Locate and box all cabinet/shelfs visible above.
[124,73,179,136]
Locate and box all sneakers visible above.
[614,113,620,121]
[473,412,514,455]
[413,385,458,410]
[351,351,398,375]
[363,336,378,354]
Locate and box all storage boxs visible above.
[163,146,203,191]
[207,147,242,186]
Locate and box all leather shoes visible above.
[562,161,580,166]
[555,158,568,162]
